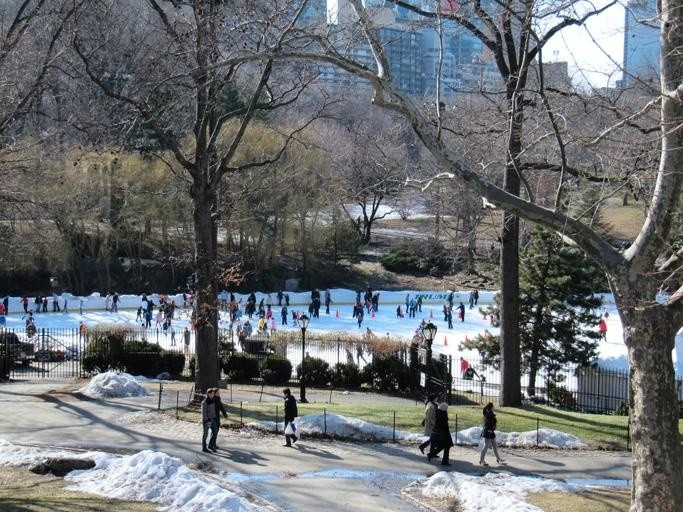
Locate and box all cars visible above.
[0,332,35,367]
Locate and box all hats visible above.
[439,403,448,411]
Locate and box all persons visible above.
[604,308,609,319]
[343,326,374,366]
[137,288,196,352]
[200,388,219,453]
[478,401,509,467]
[213,387,228,449]
[291,287,380,332]
[0,290,121,343]
[418,392,441,458]
[281,387,299,448]
[597,316,608,342]
[459,357,481,379]
[220,287,290,344]
[426,401,455,466]
[397,286,480,329]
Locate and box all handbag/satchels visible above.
[285,422,297,435]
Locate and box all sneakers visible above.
[479,461,488,466]
[497,458,505,463]
[283,437,297,446]
[203,445,218,452]
[419,446,452,465]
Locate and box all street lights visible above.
[296,312,310,403]
[421,320,437,426]
